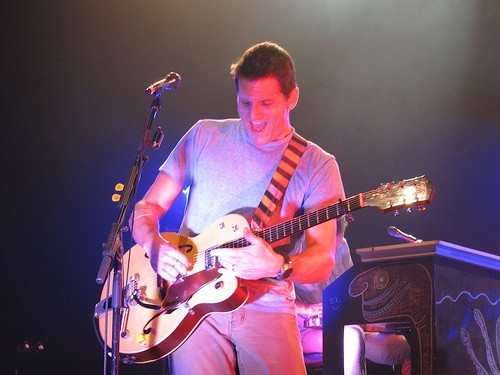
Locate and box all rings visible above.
[231,265,237,272]
[172,260,179,268]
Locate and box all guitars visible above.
[93,171,433,363]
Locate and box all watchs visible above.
[271,253,294,282]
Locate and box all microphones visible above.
[388,225,422,242]
[144,72,181,95]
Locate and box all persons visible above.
[293,215,412,375]
[128,41,346,374]
